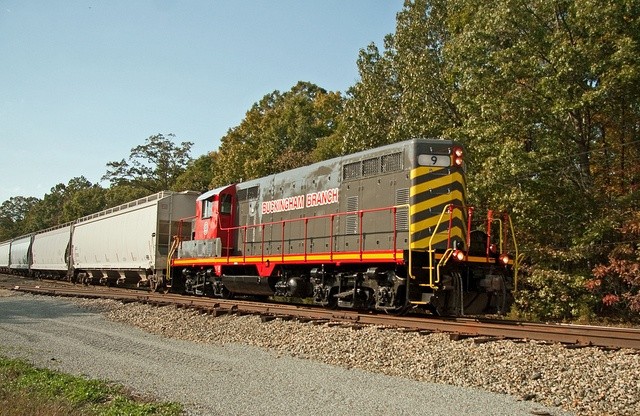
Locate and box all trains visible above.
[0,138,526,319]
